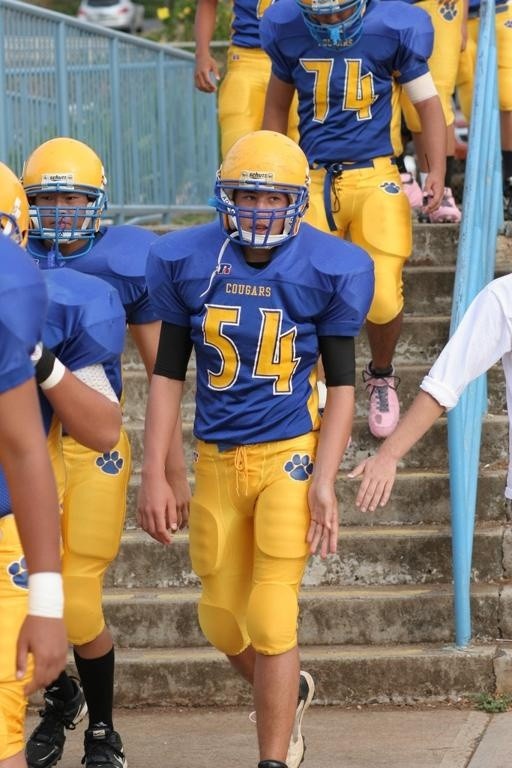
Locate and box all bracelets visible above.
[20,570,66,619]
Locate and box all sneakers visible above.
[361,357,402,441]
[81,729,128,766]
[22,675,89,768]
[419,160,512,224]
[285,669,316,768]
[399,170,424,209]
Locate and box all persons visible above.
[0,231,76,700]
[129,127,374,768]
[260,1,449,438]
[195,2,299,157]
[0,160,123,768]
[344,272,511,566]
[15,136,191,768]
[390,1,512,222]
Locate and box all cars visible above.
[74,0,146,35]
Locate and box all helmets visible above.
[22,136,108,263]
[298,1,367,52]
[0,156,29,251]
[214,129,311,248]
